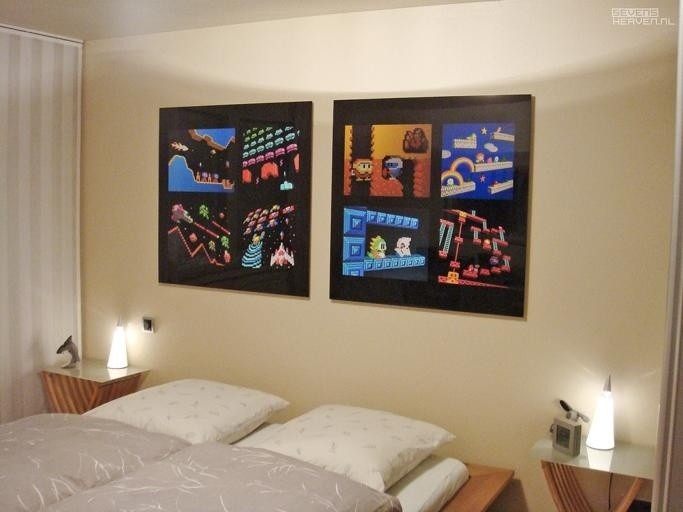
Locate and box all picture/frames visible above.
[157,99,313,297]
[329,93,531,318]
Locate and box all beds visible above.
[0,411,515,512]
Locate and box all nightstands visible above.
[37,357,150,414]
[527,424,656,511]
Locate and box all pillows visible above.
[81,376,290,443]
[231,402,457,491]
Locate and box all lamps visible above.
[107,313,129,369]
[585,374,615,450]
